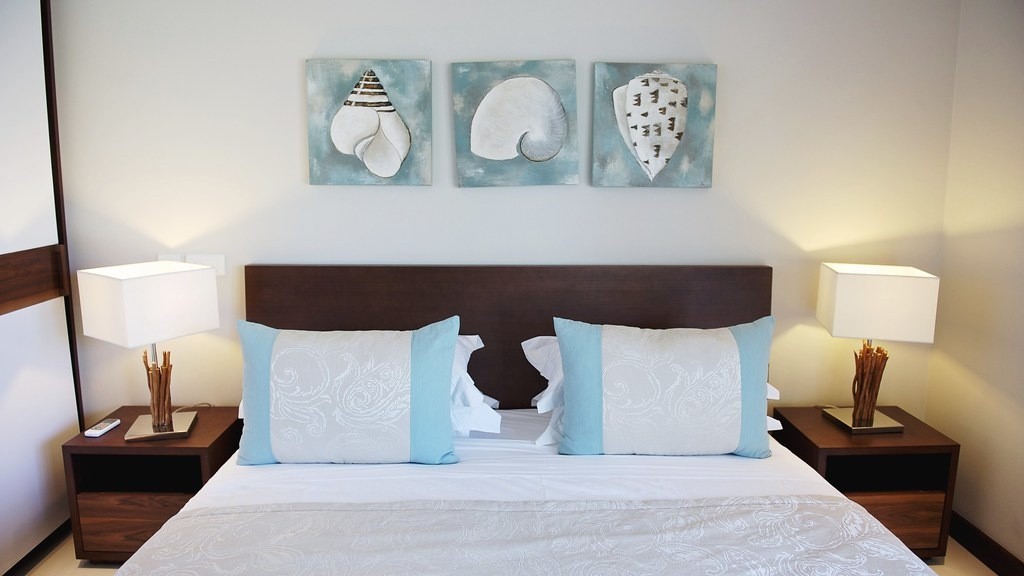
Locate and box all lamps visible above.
[77,259,221,443]
[815,262,940,436]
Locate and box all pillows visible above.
[235,316,460,466]
[236,335,502,439]
[552,314,777,459]
[520,335,783,449]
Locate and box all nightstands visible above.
[62,405,243,566]
[771,406,961,561]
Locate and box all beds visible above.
[112,263,940,576]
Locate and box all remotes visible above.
[84,419,121,438]
